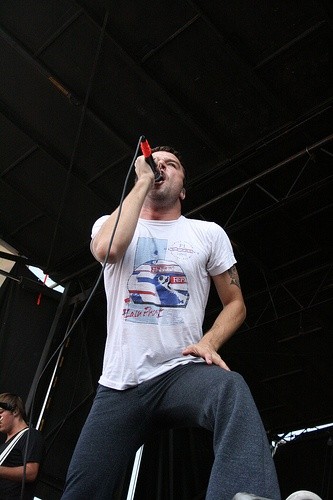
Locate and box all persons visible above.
[63,146,281,499]
[0,393,43,500]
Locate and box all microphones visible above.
[140,135,161,181]
